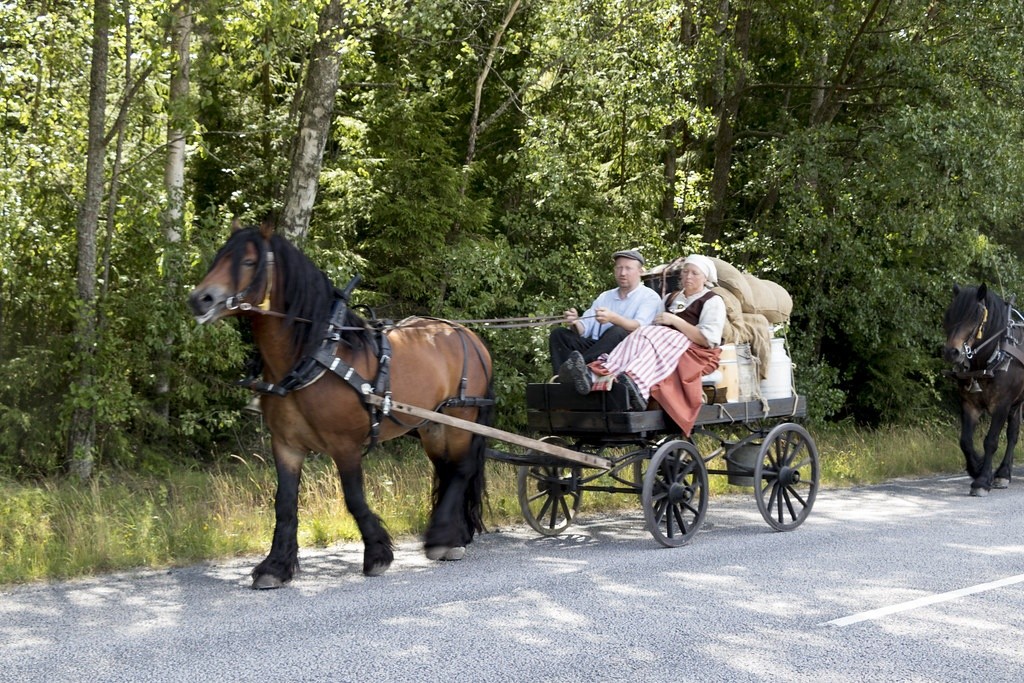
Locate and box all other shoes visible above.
[617,371,649,412]
[558,360,572,383]
[566,351,594,395]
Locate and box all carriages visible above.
[190,202,821,589]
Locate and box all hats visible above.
[612,250,644,265]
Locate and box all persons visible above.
[551,250,663,383]
[567,253,726,438]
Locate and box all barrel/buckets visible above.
[723,442,763,486]
[714,338,792,403]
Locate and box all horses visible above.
[189,211,493,589]
[943,284,1024,497]
